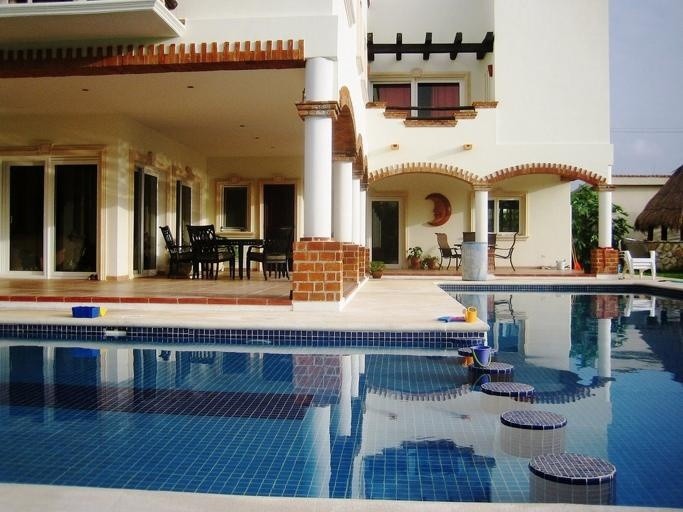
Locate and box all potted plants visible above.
[365,259,385,278]
[405,244,422,268]
[422,254,437,268]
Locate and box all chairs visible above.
[187,351,216,365]
[246,227,289,279]
[494,231,521,270]
[621,294,655,318]
[434,232,461,270]
[494,294,515,324]
[158,226,191,278]
[618,237,655,278]
[186,224,225,282]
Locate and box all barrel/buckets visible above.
[469,373,492,392]
[470,346,492,369]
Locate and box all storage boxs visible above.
[71,306,99,319]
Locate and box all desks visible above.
[215,237,267,280]
